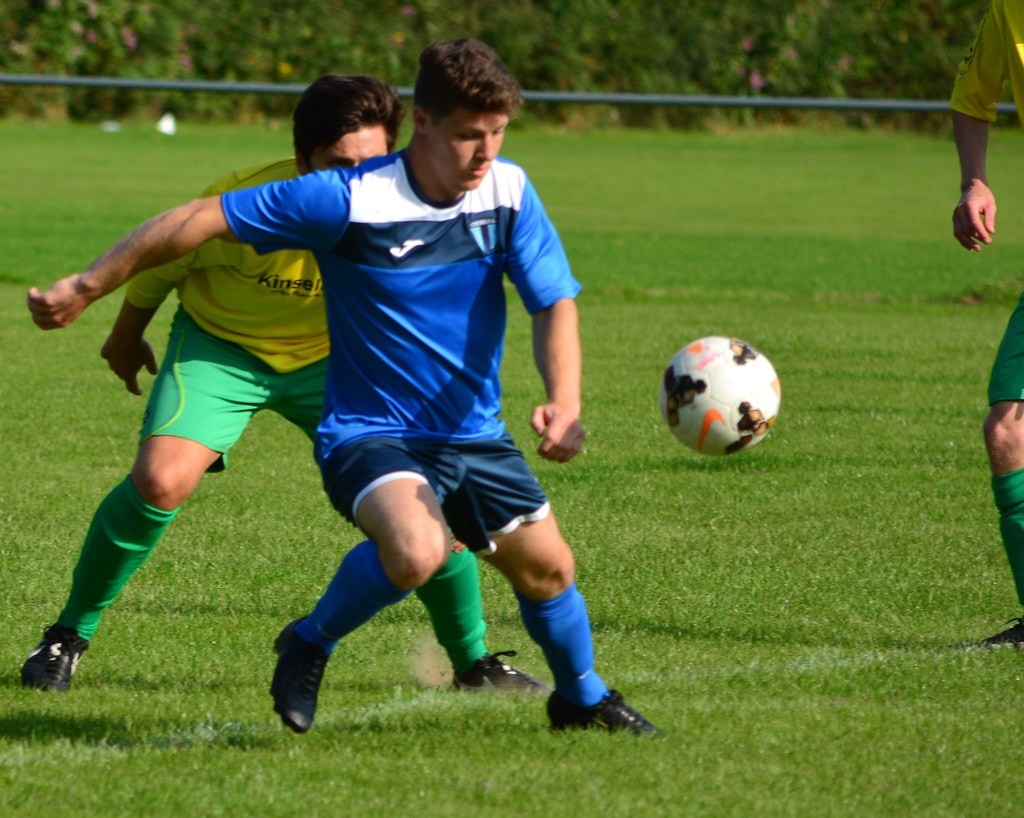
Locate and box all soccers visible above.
[657,335,782,458]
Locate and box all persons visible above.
[28,39,661,739]
[24,73,551,697]
[950,0,1024,655]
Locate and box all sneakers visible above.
[21,623,89,694]
[980,617,1024,651]
[452,650,545,691]
[269,616,328,734]
[547,689,662,733]
